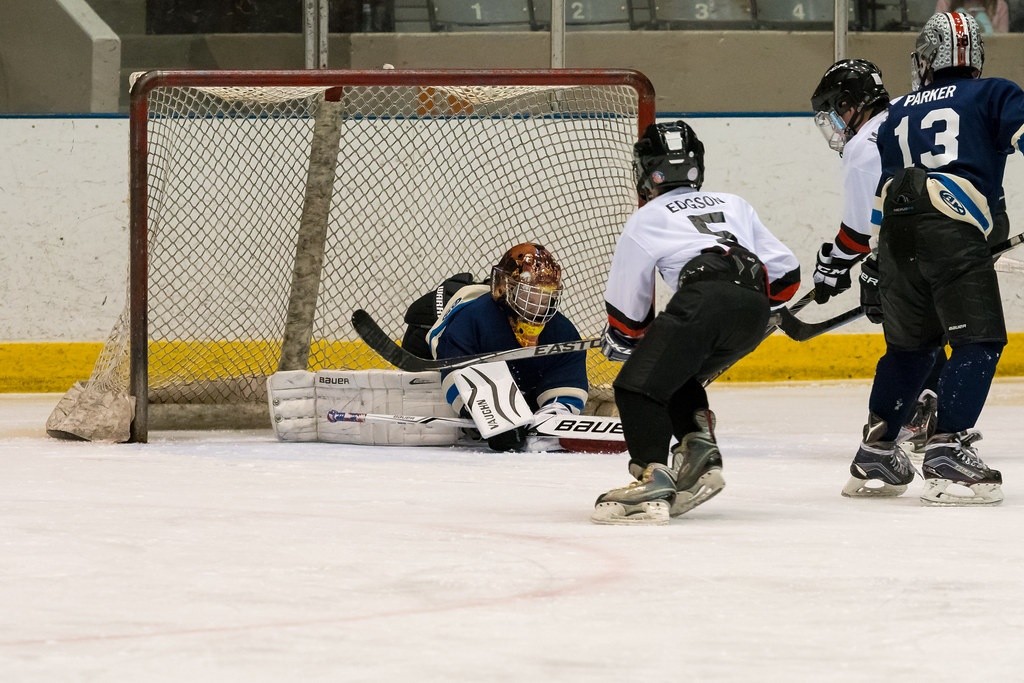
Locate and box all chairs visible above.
[391,0,940,35]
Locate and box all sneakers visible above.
[920,428,1004,507]
[840,412,915,498]
[898,389,938,464]
[670,409,725,517]
[591,458,678,527]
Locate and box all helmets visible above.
[811,58,890,114]
[915,12,984,81]
[493,242,562,300]
[633,120,704,192]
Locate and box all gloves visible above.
[487,426,527,452]
[600,321,643,363]
[813,243,869,304]
[859,255,885,324]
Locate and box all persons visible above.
[592,120,801,524]
[934,0,1009,35]
[401,242,589,451]
[810,12,1024,507]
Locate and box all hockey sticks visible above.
[327,409,626,442]
[701,251,872,388]
[351,311,782,373]
[772,233,1024,342]
